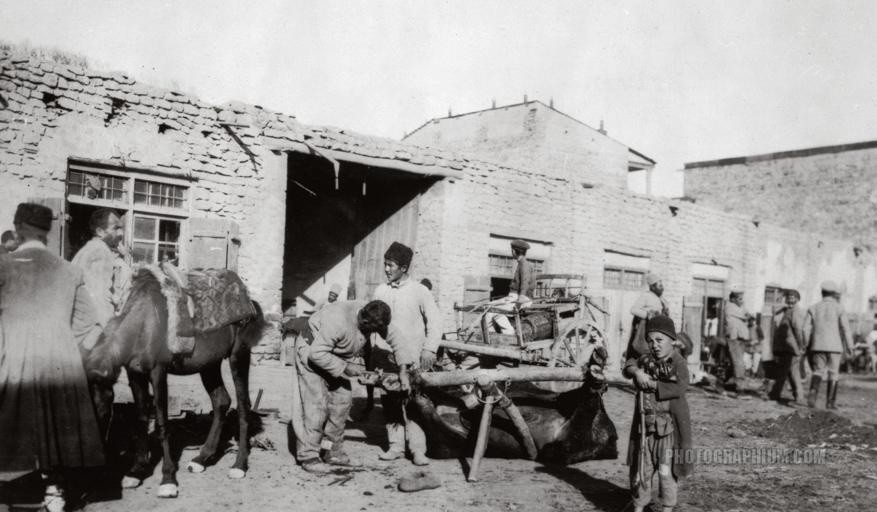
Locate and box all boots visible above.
[807,375,839,410]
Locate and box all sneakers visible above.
[299,451,350,475]
[379,447,430,466]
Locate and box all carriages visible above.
[363,271,612,420]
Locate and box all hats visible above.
[360,299,392,340]
[821,280,837,294]
[13,202,53,231]
[643,316,676,339]
[646,274,662,284]
[510,239,530,250]
[384,241,414,267]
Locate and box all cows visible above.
[347,345,619,469]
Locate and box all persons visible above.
[711,285,753,400]
[371,241,443,466]
[624,273,669,359]
[314,284,342,312]
[801,281,852,409]
[485,239,536,337]
[622,316,692,512]
[292,299,412,474]
[762,289,804,408]
[743,316,764,379]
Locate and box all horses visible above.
[81,267,265,499]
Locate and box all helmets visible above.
[786,289,801,300]
